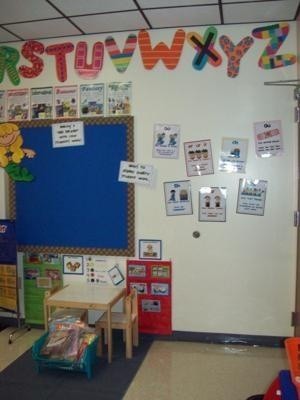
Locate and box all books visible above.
[38,307,97,363]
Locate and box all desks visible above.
[47,284,128,364]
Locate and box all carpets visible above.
[0,331,155,400]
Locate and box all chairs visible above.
[44,286,89,332]
[95,288,139,359]
